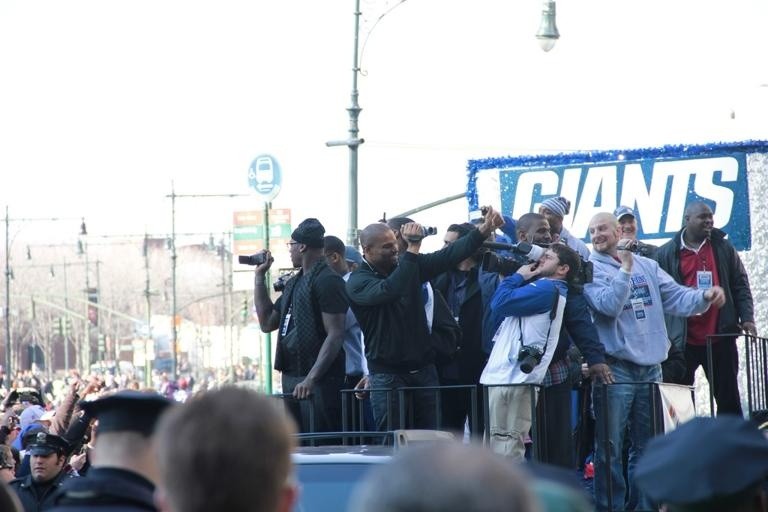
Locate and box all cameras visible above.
[420,226,437,237]
[631,243,655,255]
[238,252,265,265]
[518,346,542,374]
[273,273,293,292]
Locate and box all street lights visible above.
[325,0,559,242]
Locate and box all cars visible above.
[0,387,52,430]
[289,429,599,510]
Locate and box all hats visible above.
[80,389,178,434]
[24,433,69,456]
[291,218,325,248]
[343,246,362,263]
[542,197,570,218]
[613,207,636,221]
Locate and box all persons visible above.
[2,357,298,512]
[255,195,757,511]
